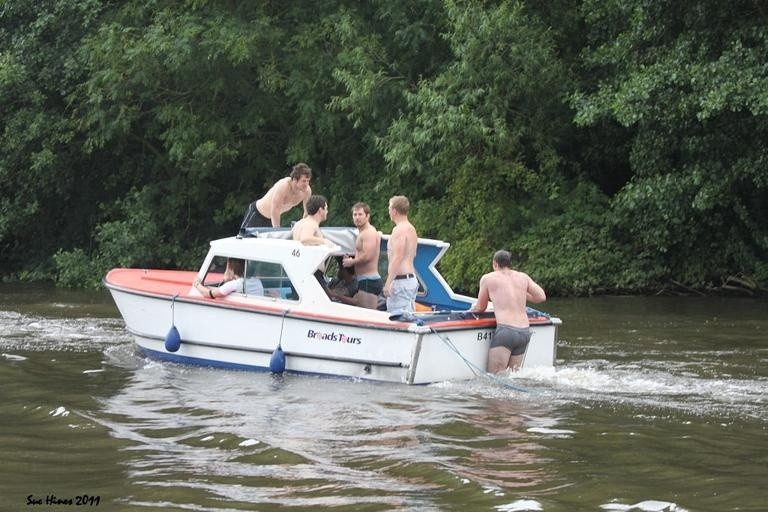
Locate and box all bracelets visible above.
[209,289,215,299]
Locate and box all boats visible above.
[100,225,563,386]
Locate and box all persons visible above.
[289,195,333,302]
[469,249,546,376]
[328,256,358,303]
[192,258,264,299]
[383,195,420,312]
[342,202,383,309]
[237,163,312,236]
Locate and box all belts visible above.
[394,274,415,280]
